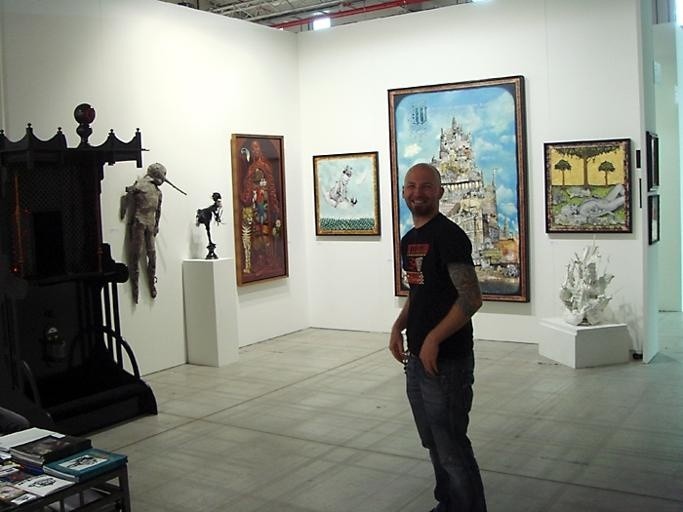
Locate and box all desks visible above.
[1,428,130,511]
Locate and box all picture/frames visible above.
[387,75,530,302]
[312,152,381,236]
[646,131,661,244]
[543,139,632,234]
[231,134,289,287]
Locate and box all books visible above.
[1,425,129,510]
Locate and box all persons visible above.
[125,162,168,304]
[237,140,280,276]
[387,161,492,511]
